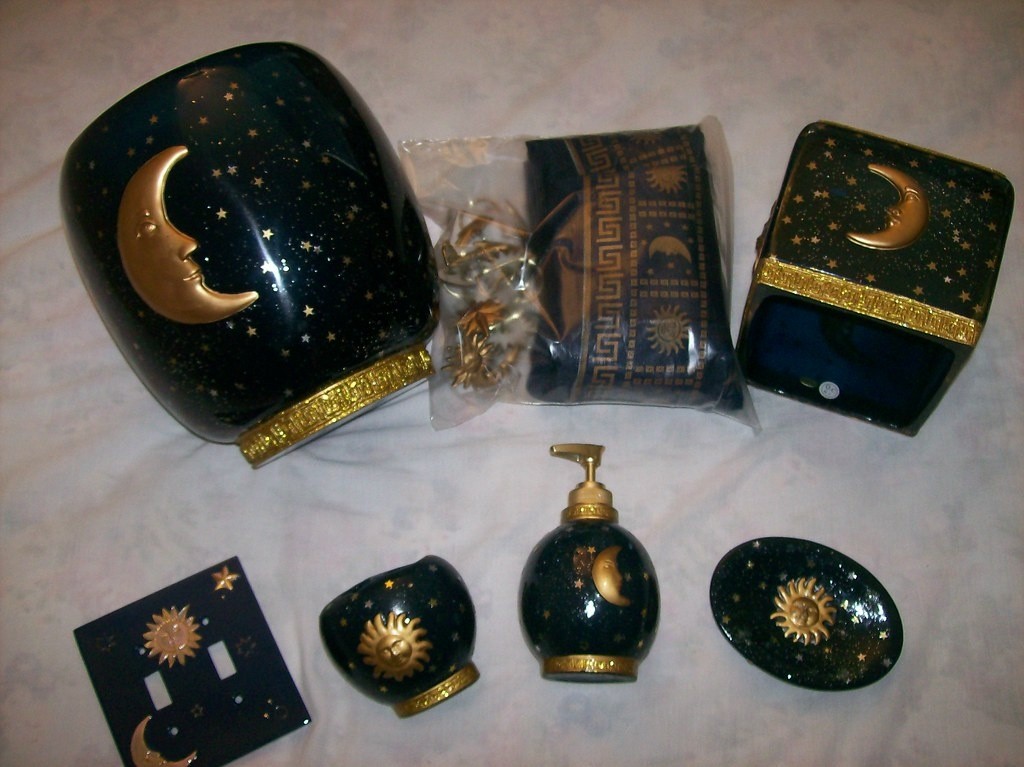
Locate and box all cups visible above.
[59,41,440,472]
[318,553,479,721]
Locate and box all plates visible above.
[709,537,903,693]
